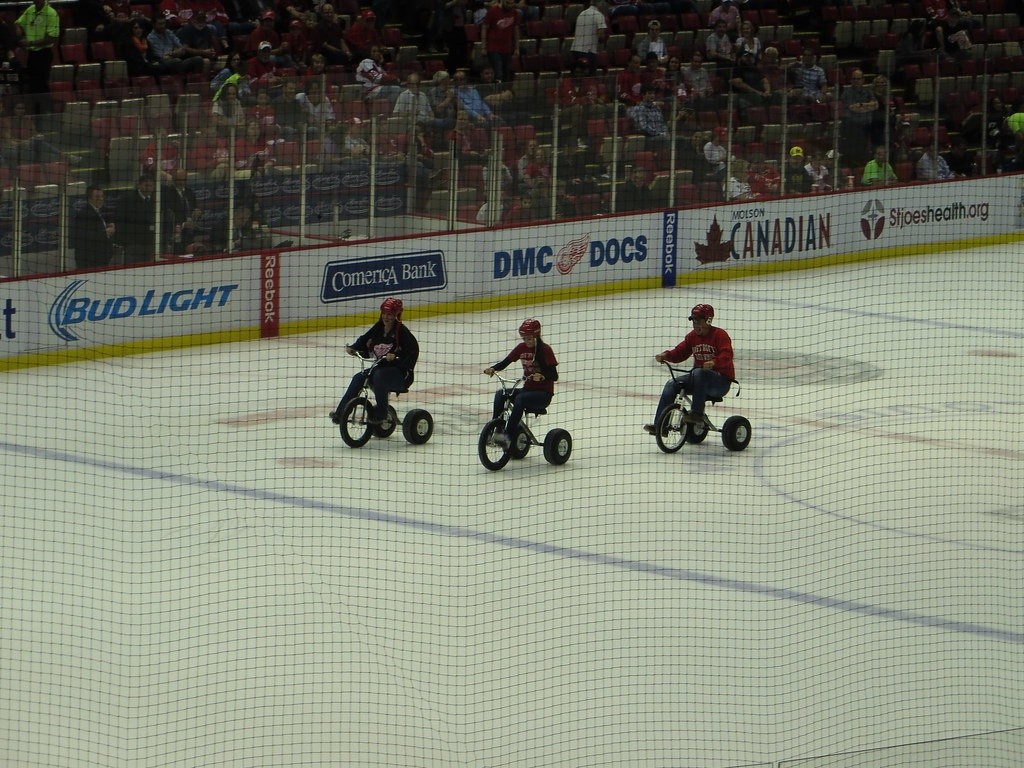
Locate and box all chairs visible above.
[0,0,1024,276]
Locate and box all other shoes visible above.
[329,411,341,424]
[368,415,388,424]
[493,433,511,449]
[65,153,83,166]
[487,434,492,441]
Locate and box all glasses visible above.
[851,77,864,80]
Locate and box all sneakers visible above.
[683,411,704,425]
[644,425,669,437]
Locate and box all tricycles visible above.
[335,344,435,448]
[650,359,753,454]
[478,370,573,470]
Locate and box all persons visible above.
[484,319,559,443]
[644,304,735,437]
[329,298,419,424]
[0,0,1024,270]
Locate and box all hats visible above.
[648,20,660,29]
[790,146,804,157]
[826,150,843,158]
[950,8,963,18]
[258,41,272,49]
[713,127,727,140]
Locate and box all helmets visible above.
[381,298,403,314]
[688,304,714,322]
[519,319,540,337]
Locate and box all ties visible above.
[145,197,150,205]
